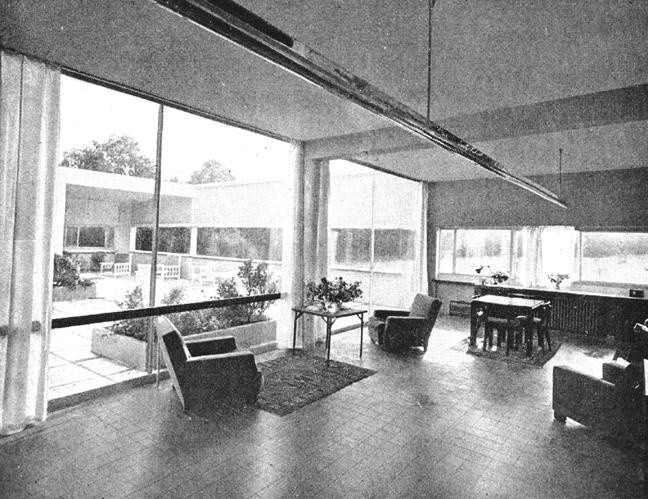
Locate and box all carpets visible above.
[254,352,379,417]
[450,333,563,369]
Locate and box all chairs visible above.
[366,294,442,353]
[469,292,551,356]
[155,315,260,413]
[156,254,181,281]
[552,363,648,447]
[99,253,133,278]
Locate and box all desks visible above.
[291,305,368,368]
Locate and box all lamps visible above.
[156,0,567,209]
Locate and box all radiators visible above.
[549,297,601,337]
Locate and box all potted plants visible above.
[305,276,362,314]
[547,272,569,290]
